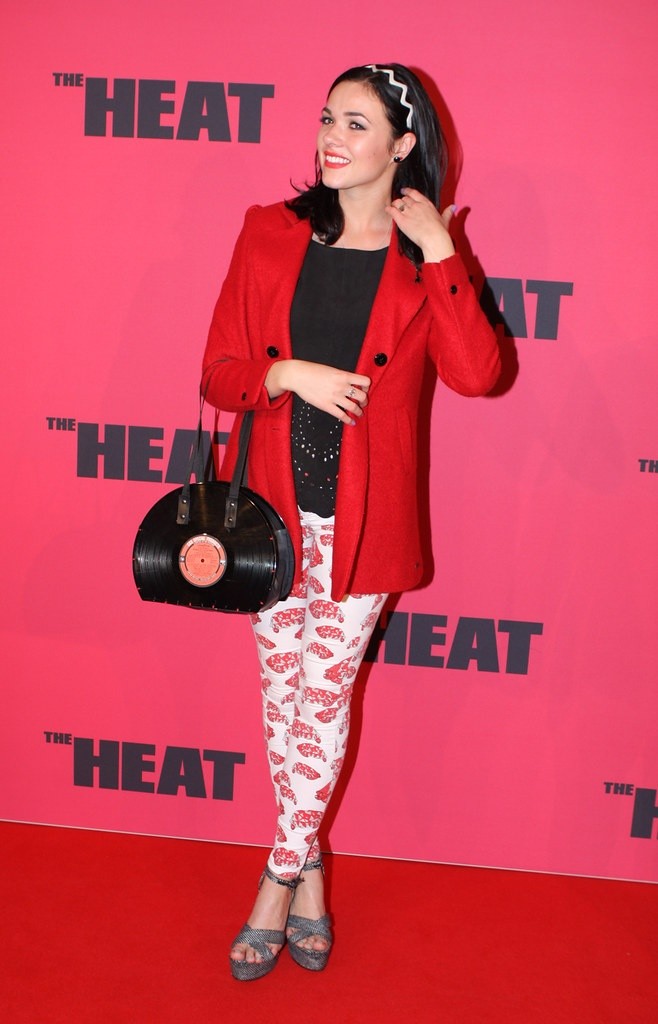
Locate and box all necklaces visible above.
[338,218,393,251]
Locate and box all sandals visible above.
[230,858,335,980]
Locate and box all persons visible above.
[199,62,502,982]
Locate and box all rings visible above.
[349,386,356,397]
[399,204,406,210]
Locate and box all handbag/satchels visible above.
[132,358,294,620]
